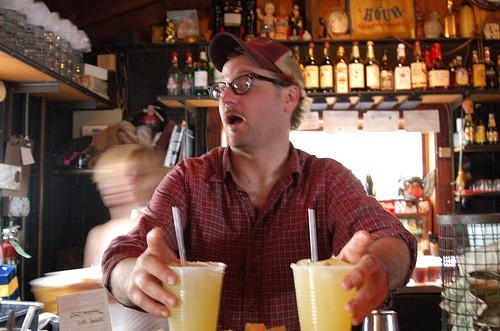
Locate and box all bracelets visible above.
[369,253,391,293]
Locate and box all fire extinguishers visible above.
[0,221,31,266]
[143,105,161,128]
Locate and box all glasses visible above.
[207,72,280,101]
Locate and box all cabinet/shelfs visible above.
[123,37,500,214]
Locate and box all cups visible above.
[469,179,500,192]
[0,7,85,87]
[162,262,227,331]
[290,263,357,331]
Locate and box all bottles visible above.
[167,0,500,143]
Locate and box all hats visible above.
[208,32,304,81]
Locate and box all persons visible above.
[101,31,419,331]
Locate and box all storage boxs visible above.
[0,300,45,331]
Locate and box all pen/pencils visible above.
[20,306,39,331]
[6,309,15,331]
